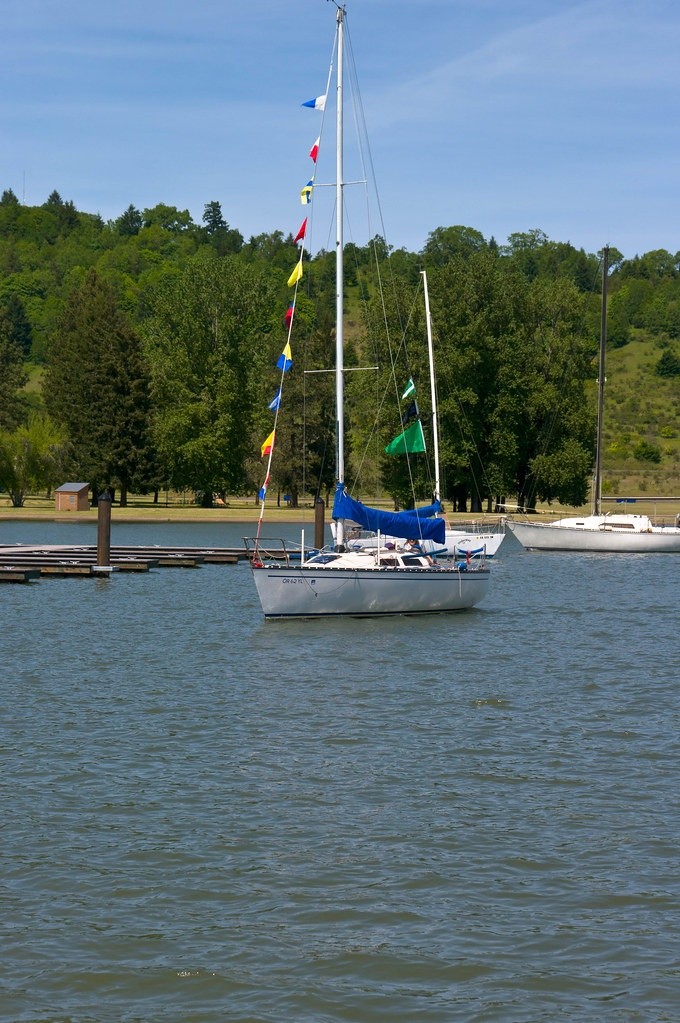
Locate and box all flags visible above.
[261,430,275,457]
[301,176,315,205]
[293,217,307,244]
[276,343,293,372]
[285,301,294,329]
[268,387,282,412]
[287,260,303,287]
[386,421,427,454]
[309,136,320,164]
[258,473,271,501]
[301,95,326,113]
[401,375,416,399]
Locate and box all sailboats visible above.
[499,246,680,551]
[348,271,508,558]
[243,0,495,618]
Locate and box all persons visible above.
[403,538,438,566]
[355,496,362,504]
[440,505,445,513]
